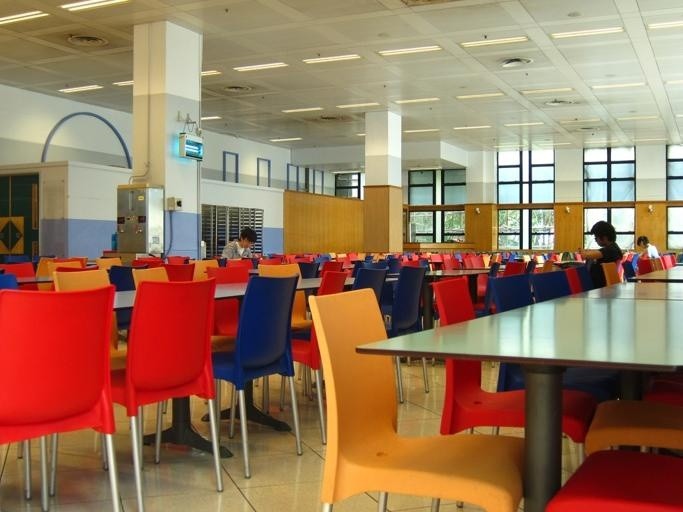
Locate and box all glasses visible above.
[243,238,256,245]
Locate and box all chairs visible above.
[212,270,304,481]
[257,248,682,433]
[428,276,584,511]
[277,267,349,445]
[1,281,124,510]
[0,250,259,472]
[307,287,525,511]
[102,275,224,511]
[544,447,683,512]
[581,397,681,460]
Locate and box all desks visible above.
[352,297,682,511]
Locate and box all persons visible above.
[637,236,660,259]
[221,228,258,260]
[578,220,623,288]
